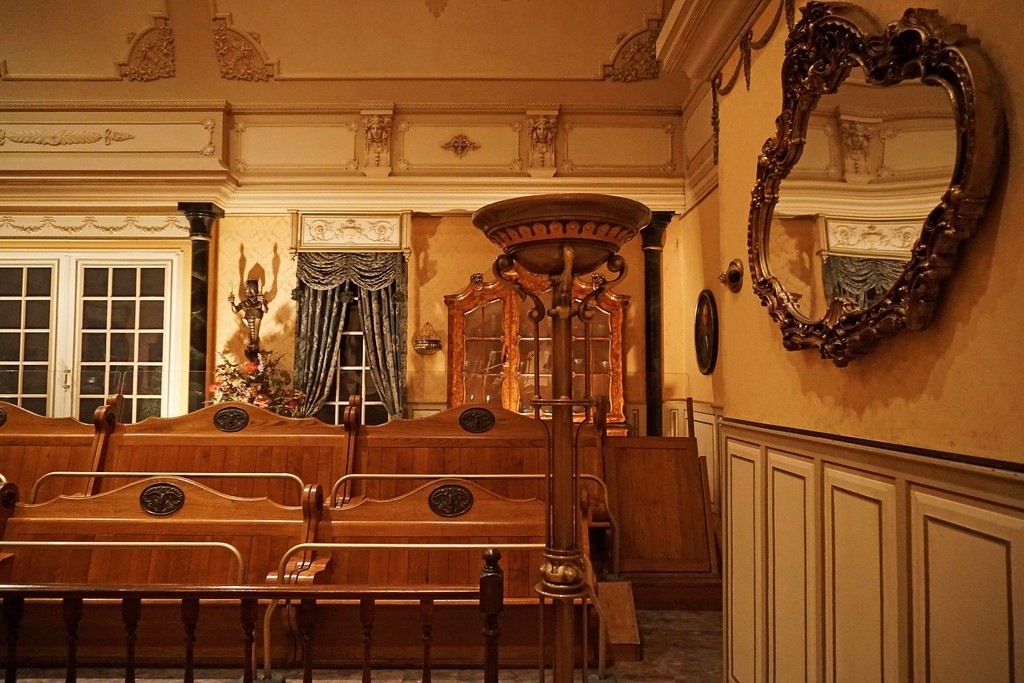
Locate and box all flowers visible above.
[200,351,307,422]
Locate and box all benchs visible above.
[0,396,723,670]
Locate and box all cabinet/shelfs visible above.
[443,264,630,438]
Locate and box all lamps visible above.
[414,321,442,356]
[472,192,653,682]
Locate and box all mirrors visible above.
[747,0,1009,370]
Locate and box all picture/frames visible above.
[694,289,718,375]
